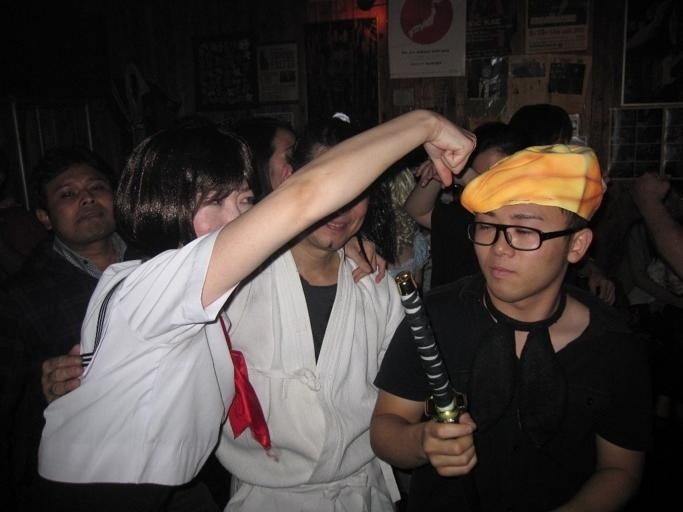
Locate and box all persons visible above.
[1,102,681,512]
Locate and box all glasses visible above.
[467,220,581,251]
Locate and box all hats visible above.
[461,142,606,222]
